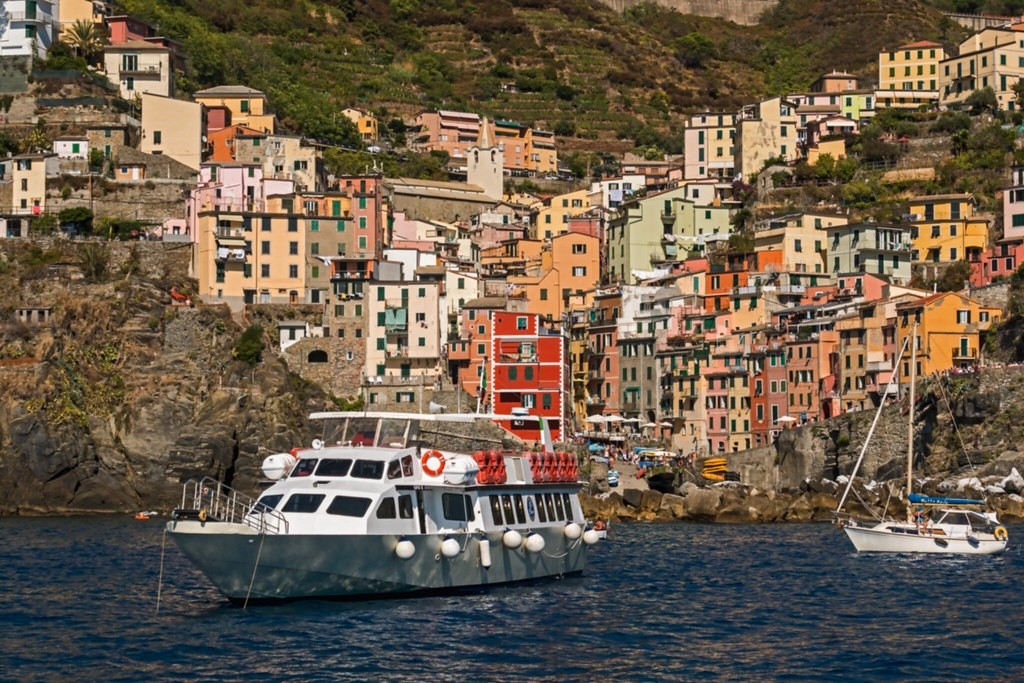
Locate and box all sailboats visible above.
[832,320,1009,552]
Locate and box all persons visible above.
[933,363,975,377]
[585,420,681,487]
[801,409,808,426]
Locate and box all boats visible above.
[167,411,606,608]
